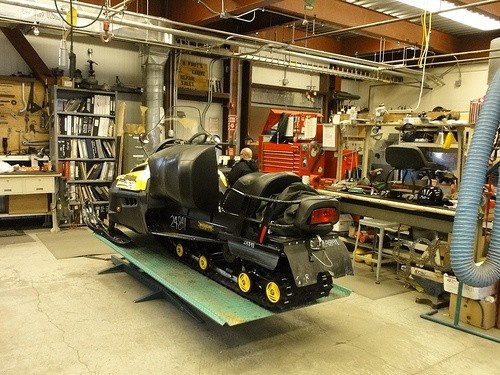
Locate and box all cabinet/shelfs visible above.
[48,86,118,230]
[0,171,62,232]
[399,122,469,191]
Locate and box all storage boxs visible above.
[443,261,497,330]
[5,194,49,214]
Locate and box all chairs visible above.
[384,145,432,200]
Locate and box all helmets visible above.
[417,185,443,205]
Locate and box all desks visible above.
[311,188,480,313]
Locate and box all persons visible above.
[228,148,259,186]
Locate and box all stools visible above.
[352,219,401,285]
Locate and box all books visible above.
[64,95,115,115]
[58,115,115,137]
[68,185,110,201]
[59,161,115,181]
[73,205,108,224]
[58,139,113,159]
